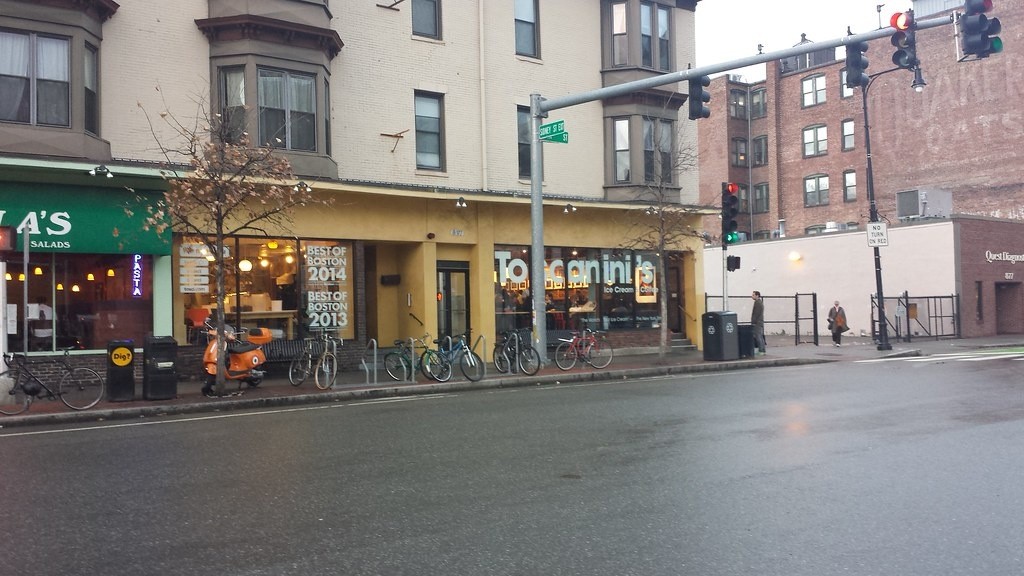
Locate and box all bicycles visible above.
[554,328,614,371]
[0,346,105,416]
[493,327,541,376]
[288,334,344,391]
[420,328,485,383]
[384,333,453,382]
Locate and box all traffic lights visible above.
[728,257,741,271]
[722,183,738,244]
[959,0,1003,58]
[890,10,917,68]
[846,42,869,88]
[689,76,711,120]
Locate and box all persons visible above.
[495,289,587,334]
[828,301,847,346]
[752,291,767,355]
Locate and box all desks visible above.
[496,310,566,329]
[226,309,298,340]
[569,307,596,329]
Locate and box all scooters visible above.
[200,317,273,395]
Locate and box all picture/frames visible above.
[519,280,526,290]
[545,275,590,290]
[502,282,509,291]
[511,281,518,291]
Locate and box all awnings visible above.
[0,180,173,253]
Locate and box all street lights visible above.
[861,59,928,350]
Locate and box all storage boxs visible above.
[249,328,273,344]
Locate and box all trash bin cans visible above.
[106,340,135,402]
[737,325,754,358]
[702,312,738,360]
[142,336,178,400]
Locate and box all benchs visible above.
[226,340,337,377]
[500,331,593,359]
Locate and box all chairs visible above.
[8,320,58,352]
[185,305,213,344]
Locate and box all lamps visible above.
[268,241,278,249]
[652,209,658,216]
[563,206,568,214]
[19,273,25,281]
[455,199,461,208]
[570,206,577,212]
[293,182,303,192]
[462,200,468,208]
[304,182,312,193]
[87,271,95,280]
[89,166,100,177]
[34,264,43,275]
[56,279,63,291]
[645,209,651,216]
[238,245,253,273]
[71,283,81,292]
[105,167,114,180]
[107,266,116,277]
[6,273,13,281]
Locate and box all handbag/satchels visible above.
[828,321,833,331]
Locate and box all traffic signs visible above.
[539,120,565,139]
[541,131,568,143]
